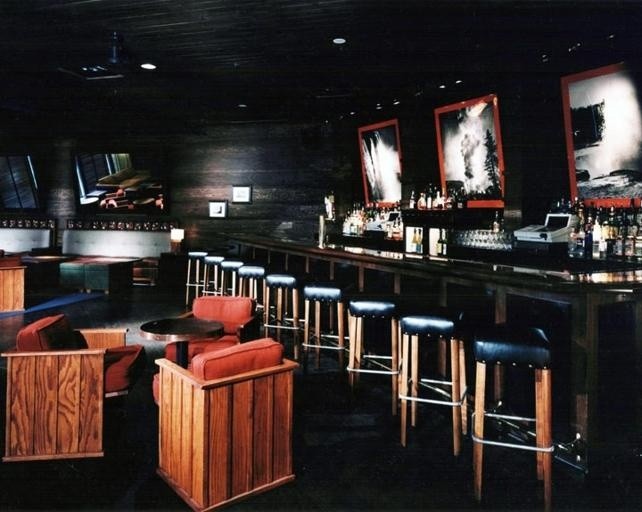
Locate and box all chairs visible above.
[100,188,164,210]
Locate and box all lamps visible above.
[170,228,185,254]
[107,30,121,65]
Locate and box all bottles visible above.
[409,228,448,256]
[493,210,506,233]
[554,197,641,259]
[410,183,466,209]
[345,203,403,242]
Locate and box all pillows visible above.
[98,174,120,184]
[114,168,132,181]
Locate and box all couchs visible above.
[95,168,143,189]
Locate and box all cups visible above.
[451,230,515,251]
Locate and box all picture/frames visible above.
[433,93,506,206]
[208,200,228,220]
[560,57,642,209]
[357,117,404,209]
[232,184,252,205]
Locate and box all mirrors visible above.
[72,150,170,215]
[0,152,42,212]
